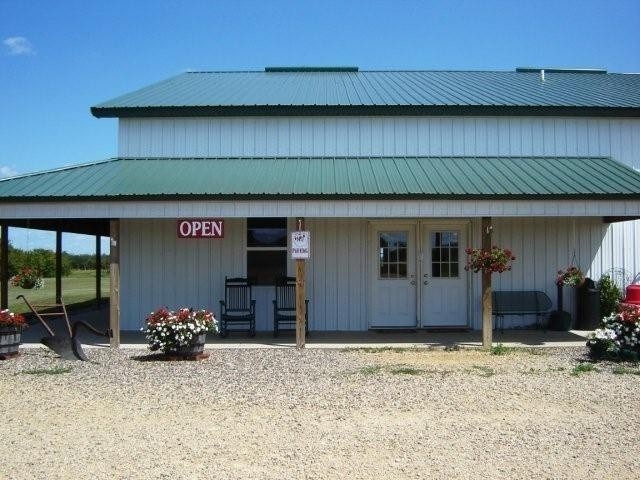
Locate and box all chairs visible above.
[219,275,309,338]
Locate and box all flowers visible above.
[554,267,586,289]
[462,246,517,276]
[138,305,221,353]
[0,309,29,332]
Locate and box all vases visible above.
[164,333,207,355]
[0,327,22,361]
